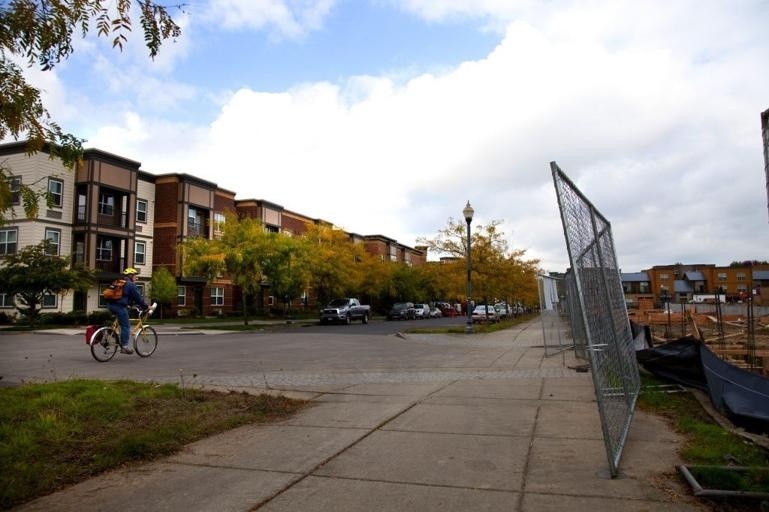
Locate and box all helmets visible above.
[123,267,139,275]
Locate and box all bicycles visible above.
[87,304,160,364]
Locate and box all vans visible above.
[700,298,719,304]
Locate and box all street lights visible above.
[461,198,476,334]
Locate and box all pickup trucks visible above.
[319,295,372,325]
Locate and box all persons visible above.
[102,267,157,354]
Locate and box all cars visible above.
[387,300,525,324]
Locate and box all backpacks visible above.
[102,279,127,301]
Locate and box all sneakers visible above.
[120,347,133,354]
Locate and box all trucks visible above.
[687,294,726,303]
